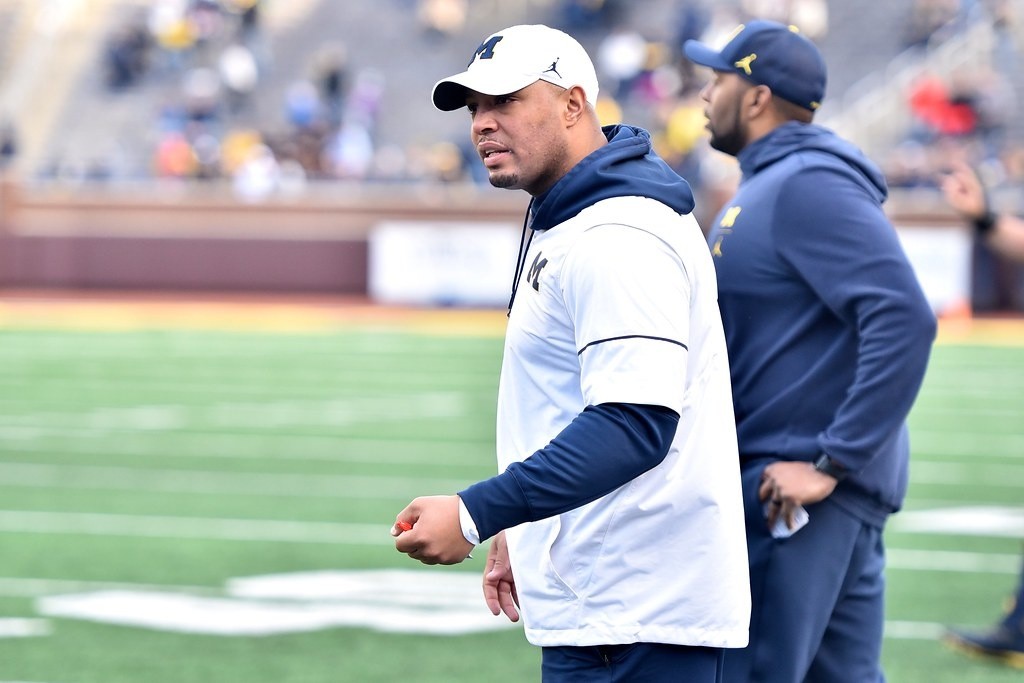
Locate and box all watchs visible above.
[812,447,847,482]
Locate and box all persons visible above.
[945,541,1024,673]
[388,24,753,679]
[682,19,940,679]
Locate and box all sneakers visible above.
[944,616,1024,672]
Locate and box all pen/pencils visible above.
[395,520,475,560]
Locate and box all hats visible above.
[679,18,833,114]
[434,22,601,117]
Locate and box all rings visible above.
[770,498,782,506]
